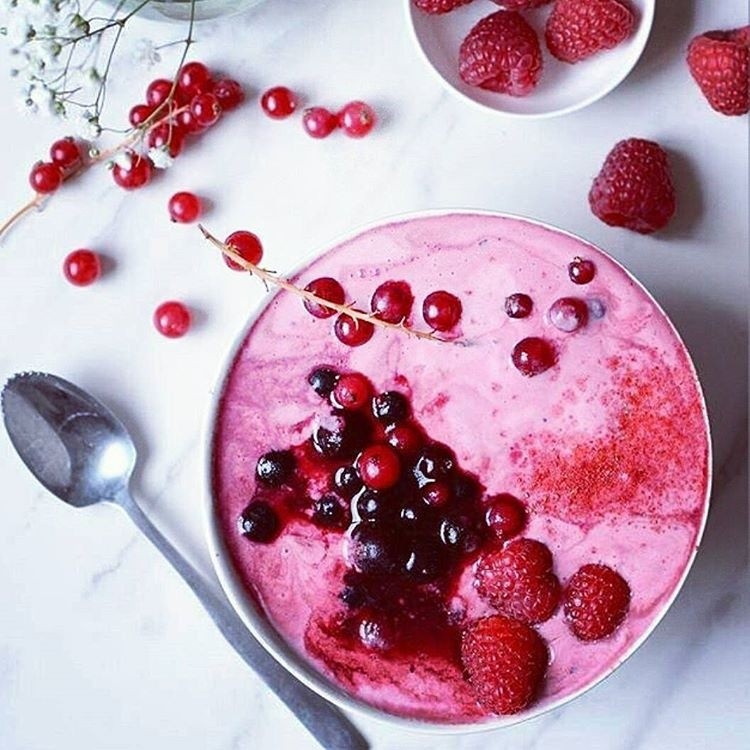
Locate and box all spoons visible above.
[0,369,373,750]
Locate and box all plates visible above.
[407,0,657,122]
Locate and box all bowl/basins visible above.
[200,209,714,736]
